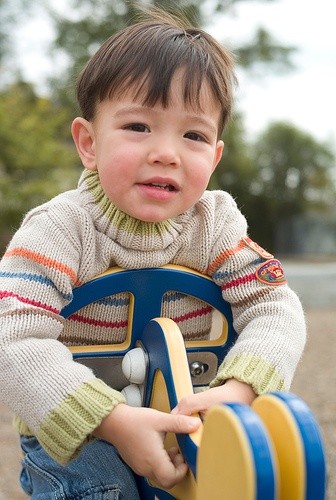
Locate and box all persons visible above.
[0,1,311,500]
